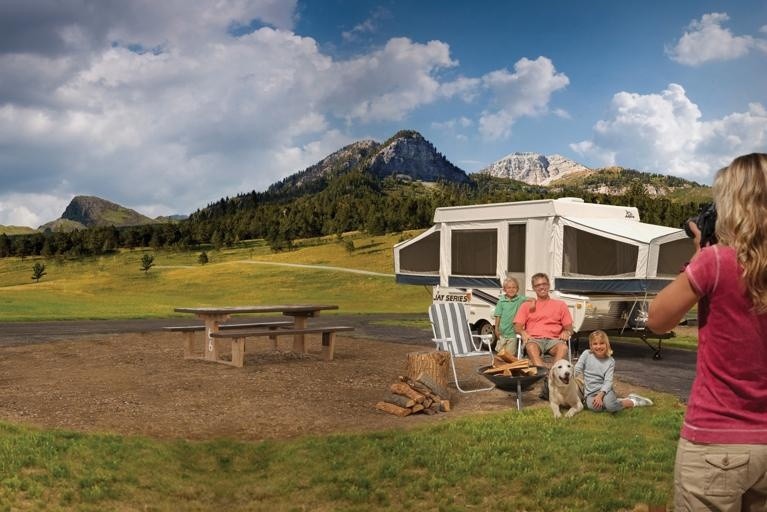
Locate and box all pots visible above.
[475,364,549,388]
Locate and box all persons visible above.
[493,276,538,365]
[573,330,655,413]
[512,272,575,382]
[646,152,767,511]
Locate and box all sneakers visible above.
[627,393,654,407]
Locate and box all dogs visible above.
[547,359,586,419]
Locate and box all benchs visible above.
[160,321,355,368]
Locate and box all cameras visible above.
[685,202,718,245]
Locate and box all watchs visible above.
[565,330,571,335]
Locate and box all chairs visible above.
[516,334,571,364]
[427,300,497,394]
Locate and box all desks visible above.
[174,304,339,362]
